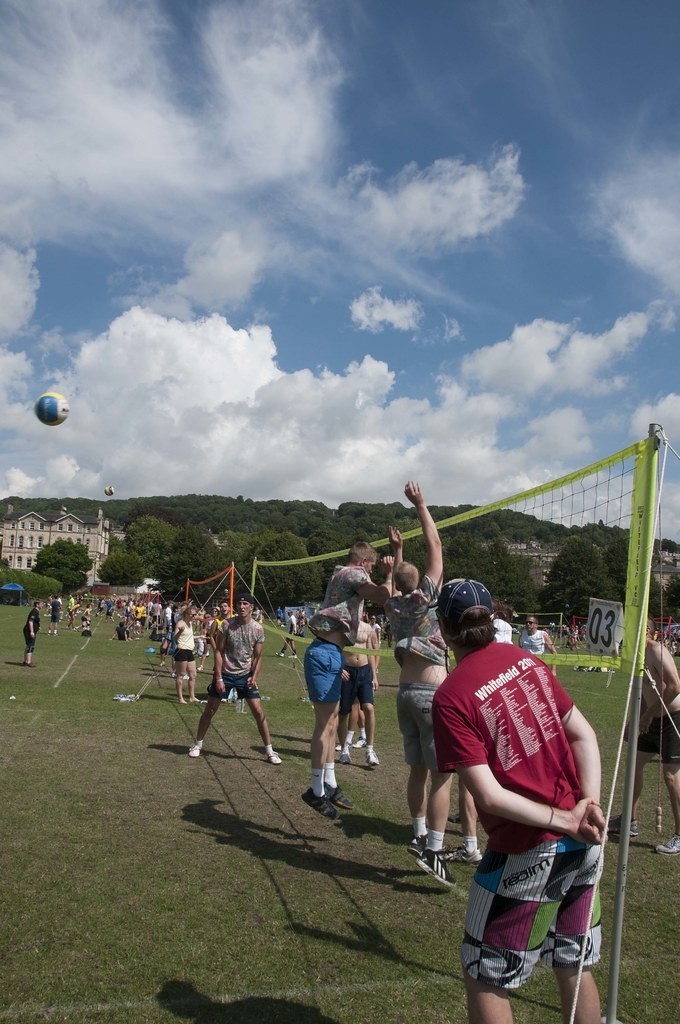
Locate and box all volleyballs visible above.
[104,486,115,497]
[34,392,70,426]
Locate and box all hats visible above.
[428,579,493,627]
[237,594,258,612]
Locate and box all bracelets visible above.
[385,579,392,584]
[216,679,223,682]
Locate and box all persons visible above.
[301,542,397,819]
[608,616,680,857]
[511,618,680,656]
[431,576,609,1024]
[518,617,558,676]
[24,598,44,667]
[491,600,514,646]
[382,482,482,888]
[47,592,393,767]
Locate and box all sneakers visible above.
[324,782,354,810]
[407,833,428,856]
[188,745,201,758]
[654,833,680,855]
[608,814,638,836]
[416,846,457,887]
[268,752,282,764]
[366,750,380,766]
[301,787,341,820]
[339,749,351,764]
[444,841,482,863]
[352,736,366,748]
[335,741,352,751]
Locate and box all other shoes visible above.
[276,652,284,657]
[288,654,297,658]
[23,661,36,668]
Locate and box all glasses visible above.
[189,613,197,617]
[525,621,538,624]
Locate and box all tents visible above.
[0,582,25,606]
